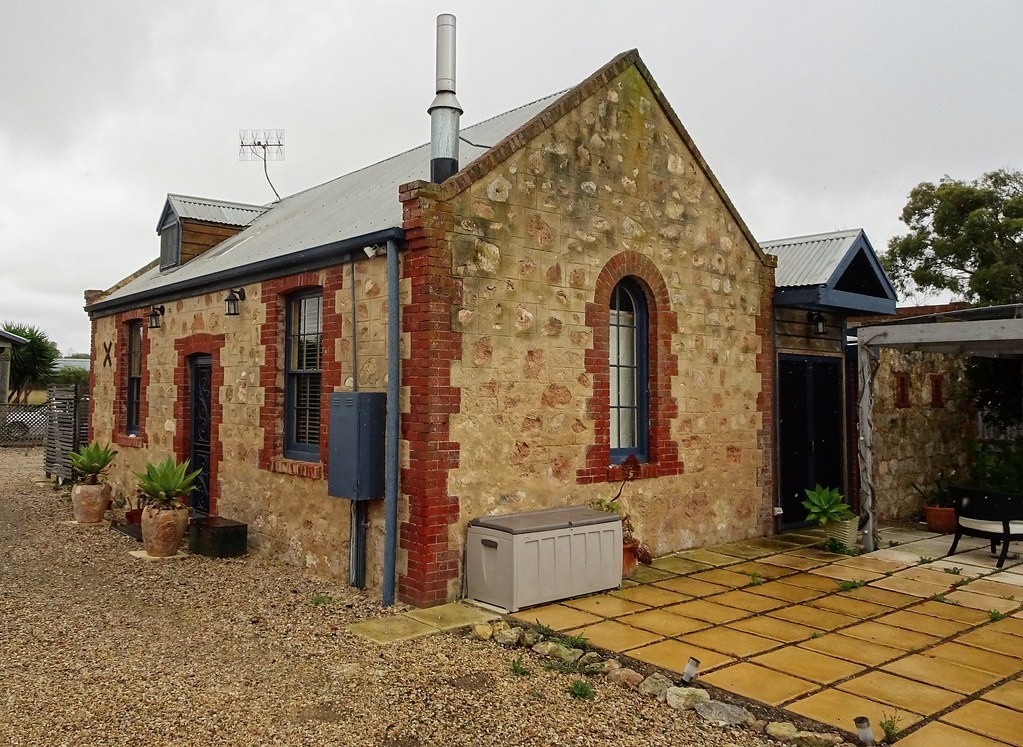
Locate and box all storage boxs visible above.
[465,504,623,614]
[187,516,250,563]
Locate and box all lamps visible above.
[222,287,247,318]
[147,305,167,329]
[807,308,828,335]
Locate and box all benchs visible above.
[947,485,1023,572]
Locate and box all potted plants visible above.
[802,482,860,550]
[592,497,652,571]
[69,440,119,523]
[133,454,205,557]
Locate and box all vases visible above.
[921,500,957,533]
